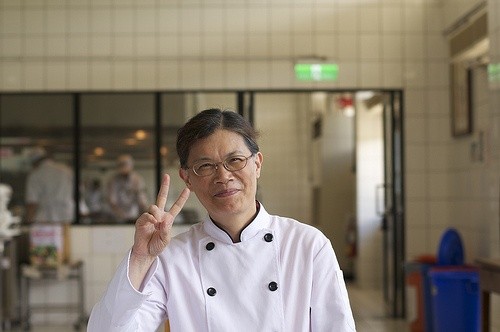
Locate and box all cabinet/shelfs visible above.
[21,261,85,332]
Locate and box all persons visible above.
[86,108,357,332]
[24,147,150,226]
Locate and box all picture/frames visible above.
[450,62,474,139]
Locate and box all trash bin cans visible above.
[405,255,434,332]
[427,266,482,332]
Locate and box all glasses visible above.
[186,153,254,177]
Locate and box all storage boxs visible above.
[29,222,70,269]
